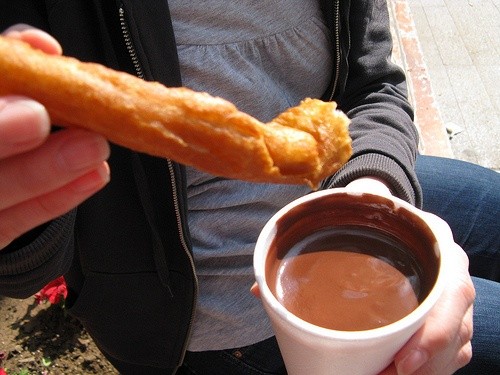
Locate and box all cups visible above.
[252,188,448,375]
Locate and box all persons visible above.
[0,0,500,375]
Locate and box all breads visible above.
[0,34,351,188]
